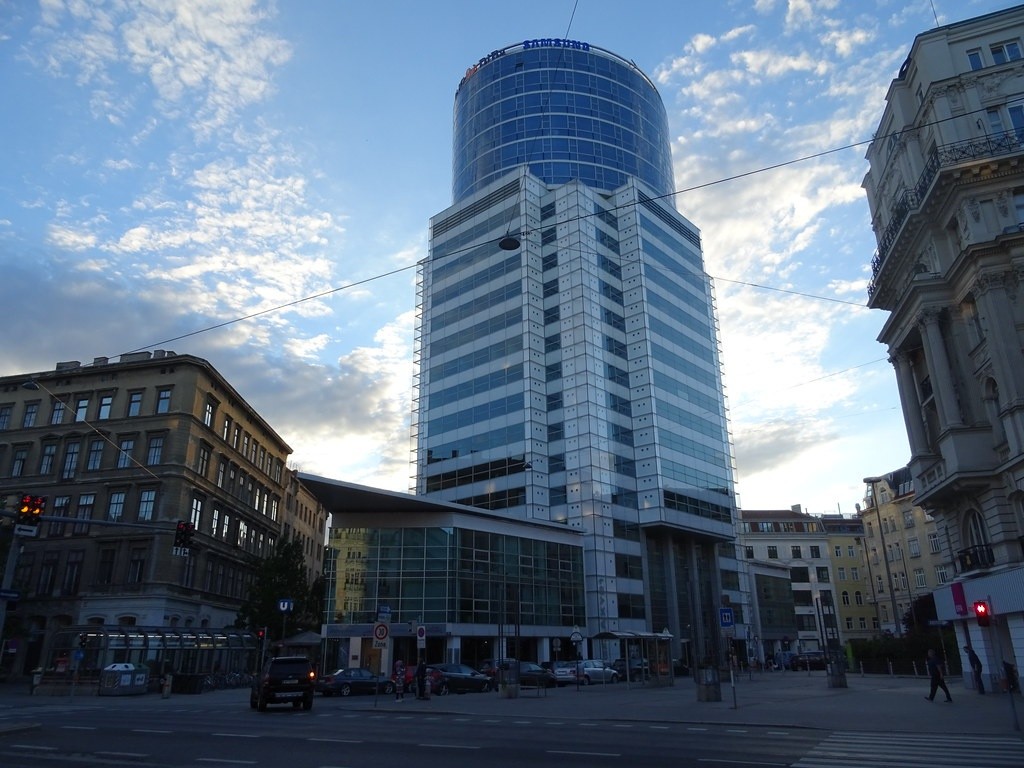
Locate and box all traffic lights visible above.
[79,633,87,647]
[257,629,265,638]
[172,519,196,548]
[974,601,989,627]
[16,493,46,524]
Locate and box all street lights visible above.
[754,635,760,674]
[864,477,903,639]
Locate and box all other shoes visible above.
[925,697,933,701]
[944,698,952,702]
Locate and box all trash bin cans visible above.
[100,663,150,696]
[32,668,44,689]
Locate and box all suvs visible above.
[249,655,318,711]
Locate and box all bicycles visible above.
[200,672,243,692]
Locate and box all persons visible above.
[578,652,583,661]
[395,660,405,703]
[924,650,953,702]
[415,660,425,700]
[964,646,985,695]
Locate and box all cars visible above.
[426,662,492,696]
[316,668,396,697]
[501,661,557,688]
[612,658,655,680]
[796,654,827,670]
[554,660,620,686]
